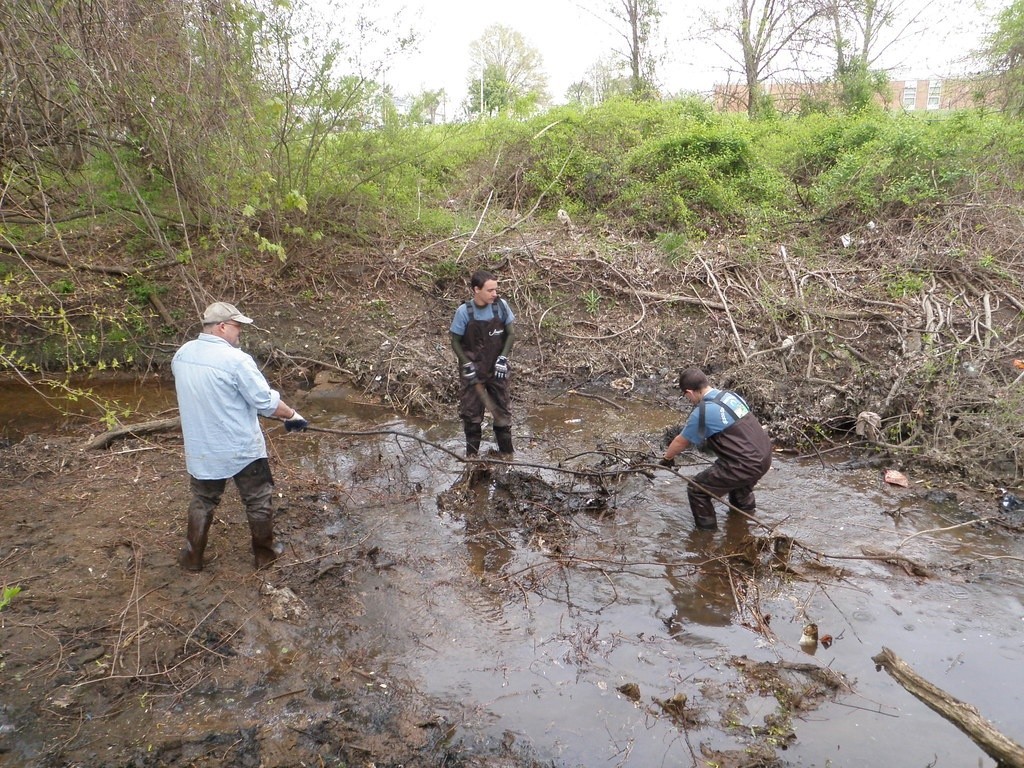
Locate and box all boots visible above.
[178,513,213,573]
[463,422,482,459]
[493,425,515,454]
[247,517,285,569]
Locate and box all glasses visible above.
[217,321,243,332]
[680,391,687,398]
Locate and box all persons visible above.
[170,302,308,571]
[450,270,516,456]
[659,367,772,529]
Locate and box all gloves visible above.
[279,408,308,432]
[658,455,677,469]
[462,361,479,386]
[494,356,508,378]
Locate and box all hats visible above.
[200,301,254,325]
